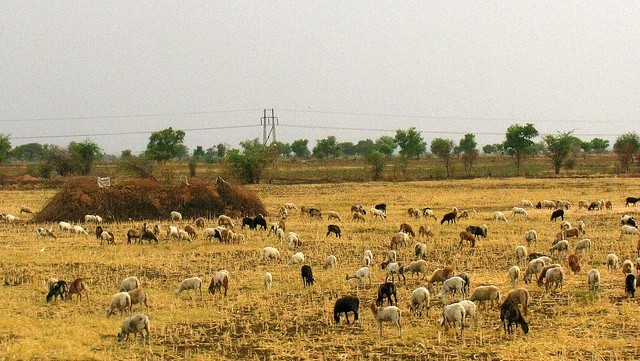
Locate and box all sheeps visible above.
[494,211,508,222]
[403,261,427,281]
[117,314,151,345]
[438,276,466,298]
[327,211,341,221]
[58,221,70,231]
[525,230,538,246]
[620,214,637,227]
[128,228,143,245]
[390,232,410,250]
[170,210,181,224]
[469,286,499,311]
[574,239,591,255]
[166,227,181,238]
[195,216,205,229]
[370,207,387,218]
[451,207,459,219]
[101,231,115,243]
[288,252,305,265]
[119,277,140,293]
[414,243,427,259]
[577,221,586,234]
[307,207,324,219]
[217,214,235,228]
[505,287,529,317]
[97,216,103,225]
[441,212,455,226]
[260,247,280,260]
[83,215,95,225]
[382,250,398,264]
[527,252,546,262]
[564,228,580,238]
[579,200,590,210]
[407,207,414,217]
[605,201,612,209]
[426,268,456,289]
[70,277,89,305]
[287,232,301,249]
[458,231,476,248]
[104,294,132,317]
[352,213,365,222]
[520,199,534,207]
[301,204,308,213]
[284,203,298,211]
[556,200,571,208]
[141,228,158,243]
[375,203,387,212]
[522,258,544,284]
[418,226,434,239]
[424,210,434,219]
[262,273,273,289]
[34,228,56,241]
[301,265,315,288]
[607,253,619,271]
[201,228,223,243]
[20,206,34,215]
[154,224,160,234]
[550,209,565,222]
[127,290,148,309]
[268,220,286,236]
[543,199,557,210]
[185,225,196,238]
[326,225,342,238]
[369,301,402,338]
[96,225,103,240]
[276,229,284,242]
[350,205,367,214]
[241,216,253,230]
[409,287,429,314]
[345,267,372,289]
[399,223,415,237]
[323,255,337,270]
[375,283,398,307]
[459,300,476,320]
[536,201,541,210]
[536,264,562,287]
[588,201,600,211]
[561,220,570,230]
[253,213,267,230]
[209,271,229,297]
[543,268,564,289]
[622,259,633,274]
[634,257,640,274]
[459,209,468,219]
[178,231,192,242]
[222,230,234,243]
[620,224,640,236]
[549,240,570,256]
[625,196,640,207]
[587,269,600,291]
[414,209,423,219]
[509,265,521,283]
[466,225,487,241]
[380,261,406,285]
[554,234,564,244]
[47,280,68,303]
[567,255,581,273]
[510,206,529,217]
[440,303,467,333]
[174,278,201,301]
[363,249,374,266]
[67,226,87,237]
[333,296,360,323]
[236,234,243,244]
[457,272,470,295]
[516,246,527,263]
[5,214,20,222]
[501,301,529,335]
[624,274,637,298]
